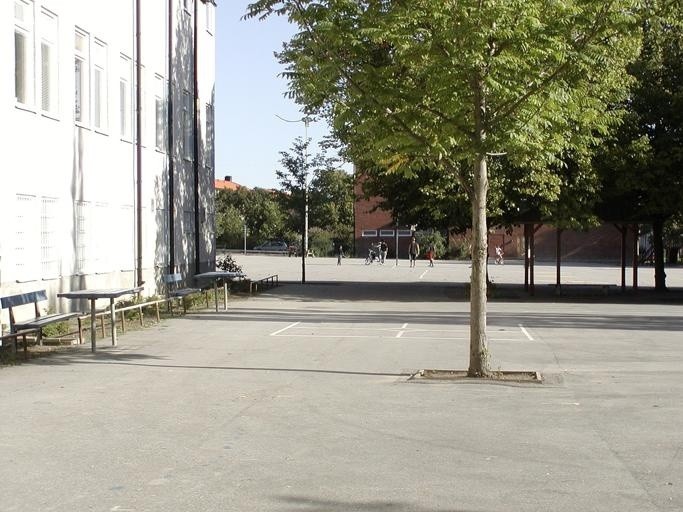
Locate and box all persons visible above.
[494,245,504,260]
[378,238,388,264]
[407,236,419,268]
[335,245,346,266]
[367,242,376,260]
[425,241,435,267]
[373,241,382,262]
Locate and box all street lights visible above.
[300,116,314,258]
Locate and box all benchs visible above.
[0,287,87,360]
[159,270,200,314]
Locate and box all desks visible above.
[194,269,239,313]
[58,283,143,353]
